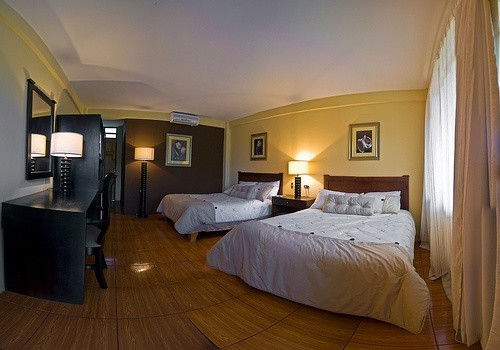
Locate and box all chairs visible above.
[84,172,118,289]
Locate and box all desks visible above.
[3,189,102,304]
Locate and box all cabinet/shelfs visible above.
[55,114,105,220]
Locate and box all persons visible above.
[172,141,186,161]
[256,140,262,155]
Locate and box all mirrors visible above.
[25,78,57,180]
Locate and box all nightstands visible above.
[271,195,316,218]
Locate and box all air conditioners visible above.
[170,112,201,126]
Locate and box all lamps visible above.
[287,160,309,197]
[50,132,83,192]
[29,133,47,172]
[134,147,154,218]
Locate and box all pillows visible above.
[239,181,258,186]
[258,180,280,199]
[322,194,374,216]
[256,185,275,202]
[360,191,402,214]
[310,188,364,210]
[230,184,258,200]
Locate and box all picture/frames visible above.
[250,132,267,161]
[165,133,192,168]
[347,122,380,161]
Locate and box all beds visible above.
[156,171,284,242]
[206,174,432,334]
